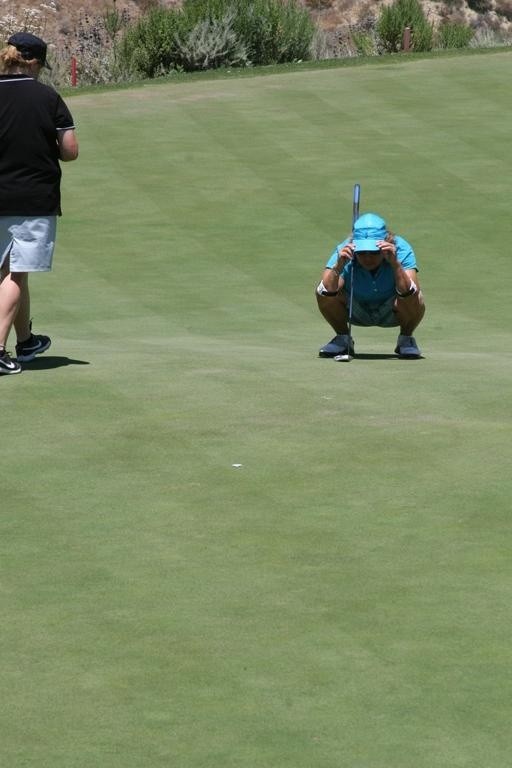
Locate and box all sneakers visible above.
[394,335,421,358]
[319,334,355,359]
[0,352,22,375]
[16,321,52,362]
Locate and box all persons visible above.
[0,32,79,374]
[316,213,426,358]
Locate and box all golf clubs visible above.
[334,184,360,362]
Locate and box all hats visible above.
[8,31,53,70]
[351,213,387,256]
